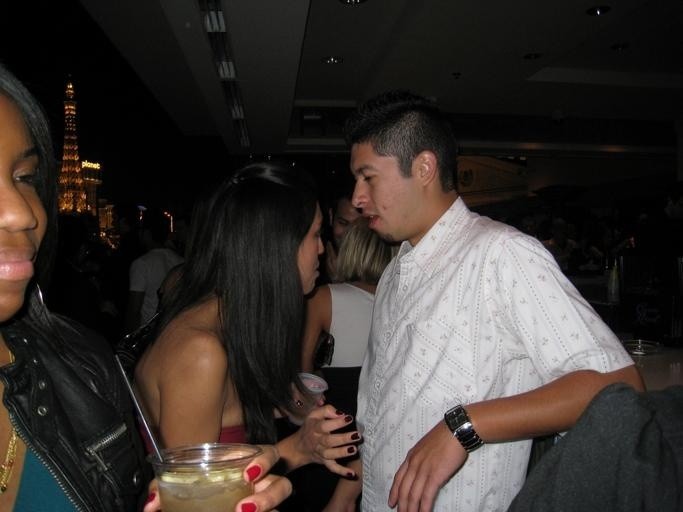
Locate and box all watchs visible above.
[441,406,483,452]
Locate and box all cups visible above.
[283,372,329,428]
[148,440,264,512]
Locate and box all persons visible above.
[120,212,184,337]
[1,68,293,512]
[326,176,361,248]
[127,163,361,479]
[321,86,635,512]
[294,216,396,378]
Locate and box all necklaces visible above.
[0,344,22,499]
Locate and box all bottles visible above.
[606,258,620,304]
